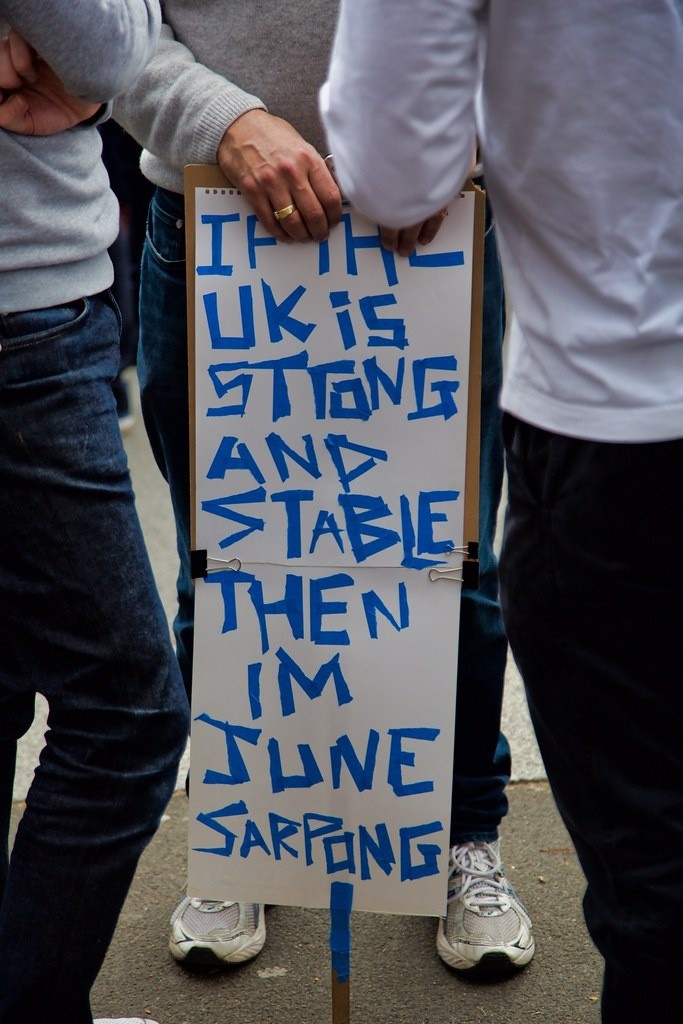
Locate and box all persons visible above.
[108,0,538,983]
[0,0,190,1024]
[314,0,683,1024]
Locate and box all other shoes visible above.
[91,1017,159,1024]
[112,380,135,429]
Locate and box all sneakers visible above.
[437,826,535,984]
[169,881,276,977]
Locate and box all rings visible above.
[274,205,296,220]
